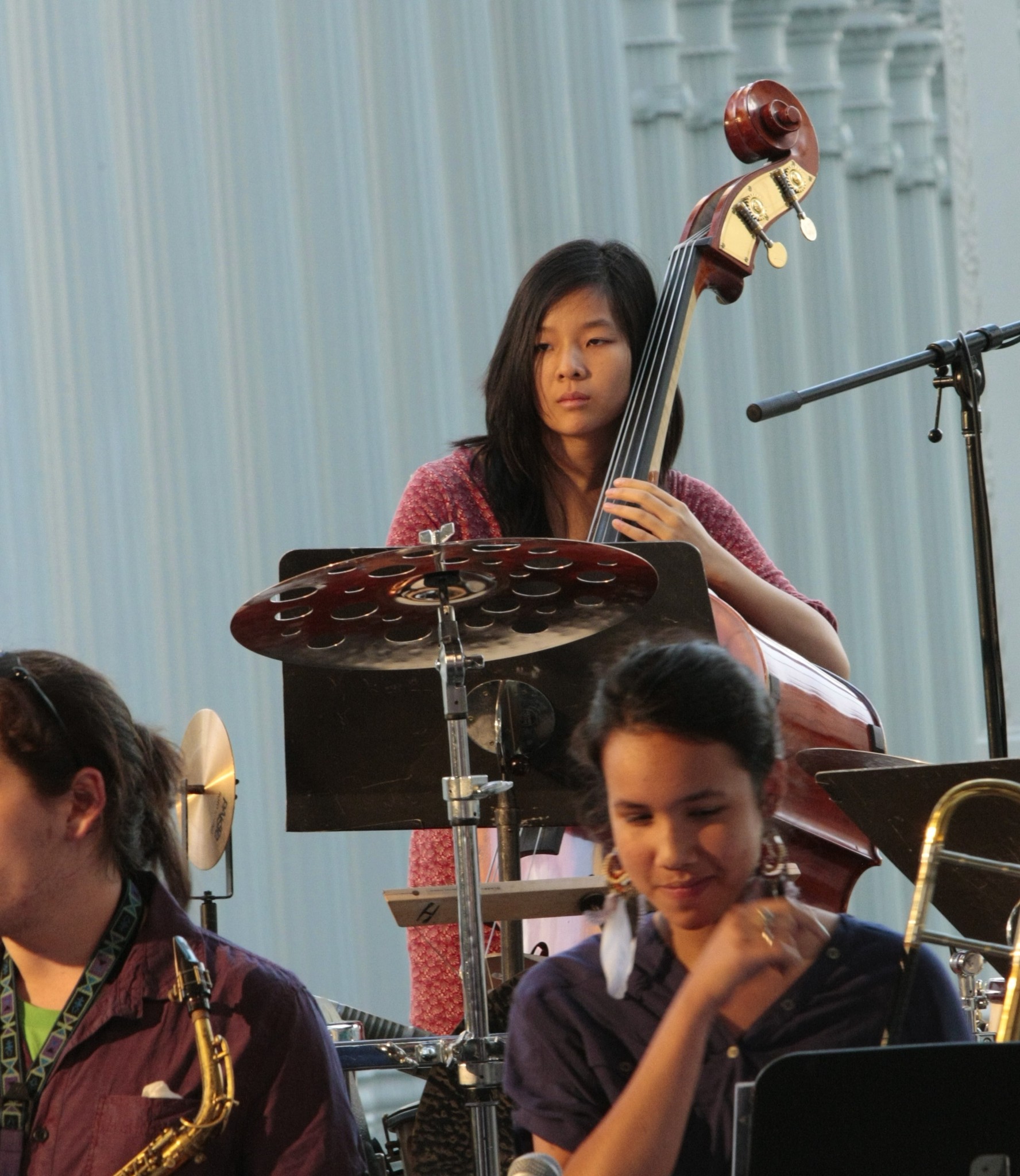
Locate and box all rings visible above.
[762,927,775,947]
[756,907,777,925]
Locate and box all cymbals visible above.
[228,533,664,680]
[795,744,929,778]
[172,706,238,871]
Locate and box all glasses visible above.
[0,650,91,766]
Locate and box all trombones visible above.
[881,778,1020,1054]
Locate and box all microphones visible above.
[507,1151,562,1176]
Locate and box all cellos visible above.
[509,71,890,975]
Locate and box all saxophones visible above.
[108,933,240,1176]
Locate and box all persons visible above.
[387,239,851,1036]
[504,642,975,1176]
[0,651,368,1176]
